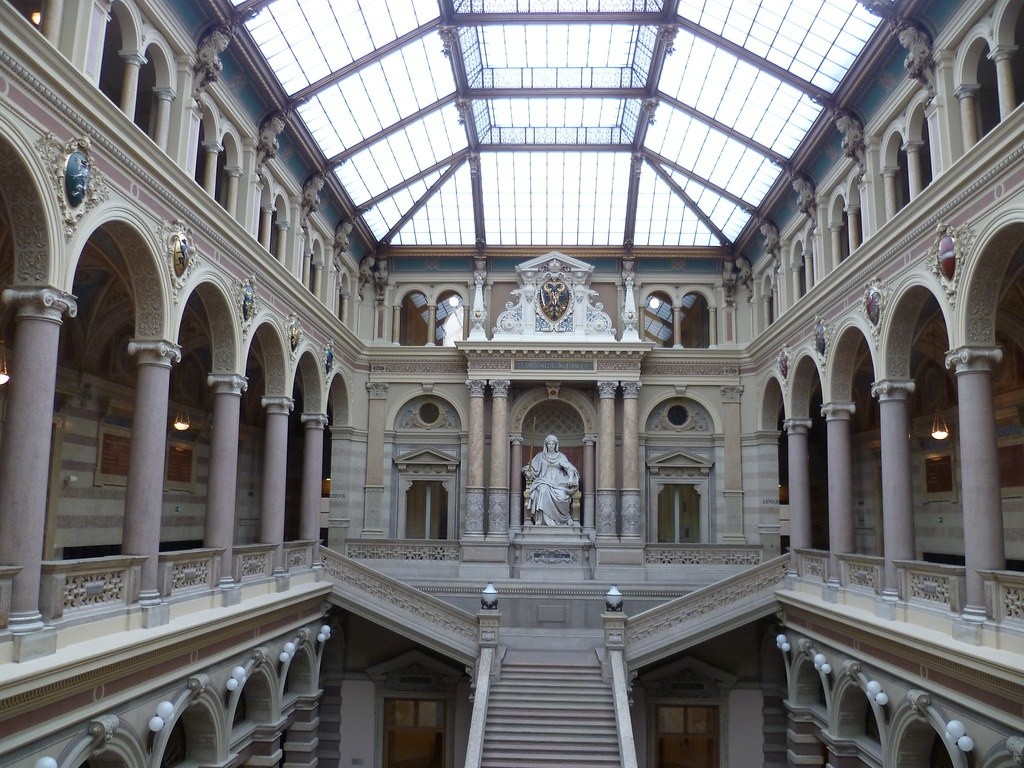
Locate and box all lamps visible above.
[223,667,247,709]
[815,654,832,694]
[931,308,948,439]
[174,327,191,431]
[315,625,332,654]
[277,642,296,677]
[0,339,11,386]
[776,634,792,666]
[34,757,57,768]
[866,681,891,724]
[147,701,174,753]
[606,584,624,611]
[945,721,975,768]
[480,581,500,609]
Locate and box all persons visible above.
[358,257,375,291]
[256,117,286,175]
[760,224,781,269]
[623,261,636,287]
[474,260,487,286]
[193,31,230,100]
[899,27,936,102]
[521,434,580,526]
[375,260,388,303]
[333,223,353,266]
[833,114,867,178]
[792,178,817,229]
[736,257,754,303]
[300,177,325,232]
[723,262,736,305]
